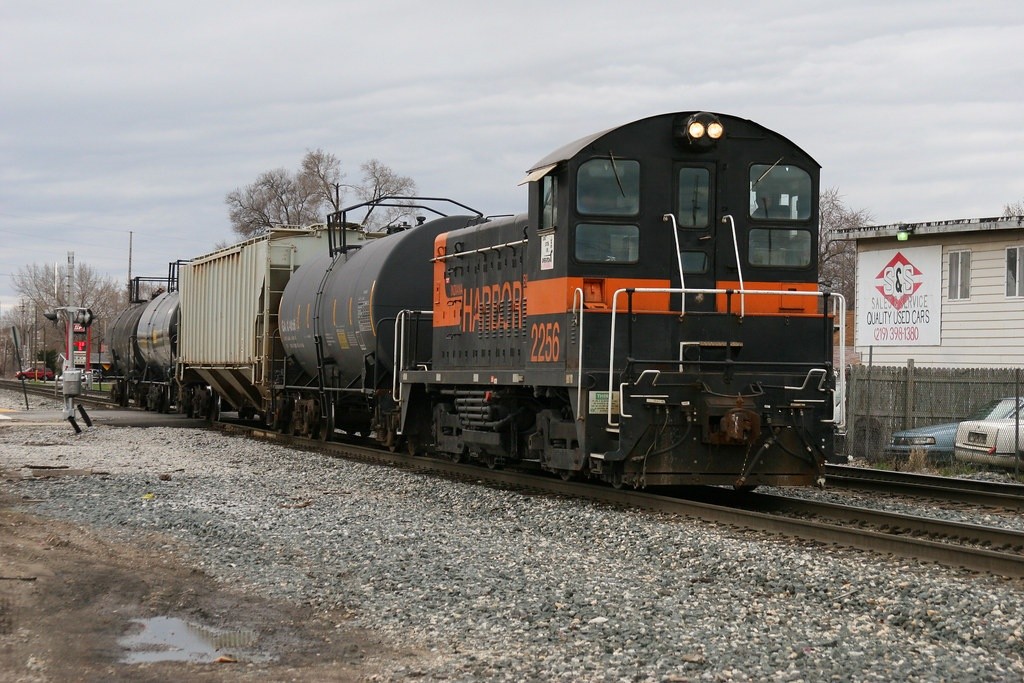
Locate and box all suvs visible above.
[15,367,54,381]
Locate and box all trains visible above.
[108,112,845,498]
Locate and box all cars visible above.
[60,368,99,381]
[888,397,1024,471]
